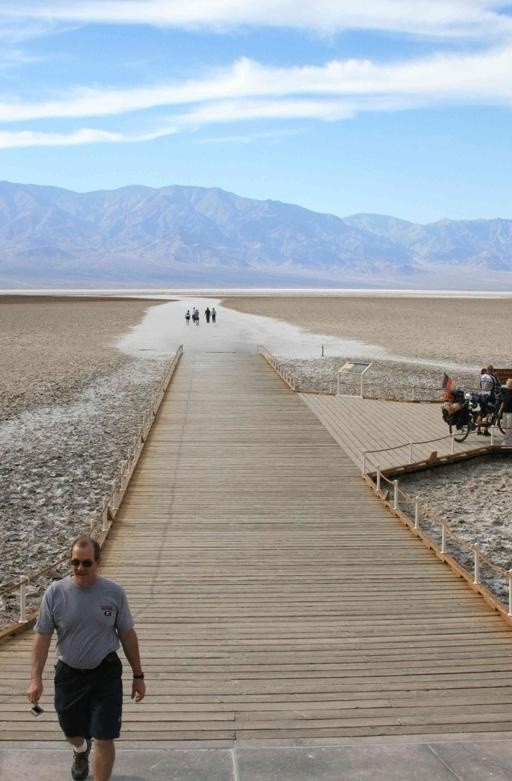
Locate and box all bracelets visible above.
[132,670,144,679]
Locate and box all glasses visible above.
[71,560,98,568]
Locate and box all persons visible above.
[191,306,196,323]
[477,364,500,436]
[194,309,199,325]
[211,307,217,322]
[204,306,210,322]
[501,377,512,428]
[27,534,145,780]
[185,308,190,325]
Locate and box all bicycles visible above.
[450,394,512,442]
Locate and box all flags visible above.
[443,372,455,401]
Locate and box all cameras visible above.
[30,705,44,715]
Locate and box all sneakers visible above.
[477,430,490,435]
[71,741,91,780]
